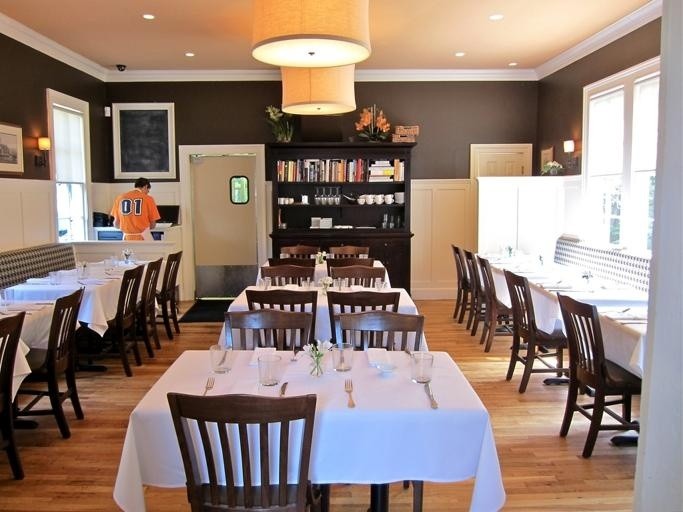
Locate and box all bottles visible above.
[313,186,340,206]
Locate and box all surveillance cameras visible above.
[117,65,126,71]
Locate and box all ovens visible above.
[97,230,163,241]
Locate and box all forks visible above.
[344,379,355,408]
[201,377,215,396]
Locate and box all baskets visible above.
[392,134,416,142]
[395,125,419,135]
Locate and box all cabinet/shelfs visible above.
[262,141,417,233]
[268,234,414,297]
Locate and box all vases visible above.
[549,167,559,176]
[310,357,323,376]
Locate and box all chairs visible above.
[452,243,515,352]
[13,286,85,440]
[155,250,183,340]
[137,257,163,358]
[1,310,26,481]
[82,264,145,377]
[557,290,641,459]
[164,392,316,511]
[504,268,571,394]
[223,245,428,352]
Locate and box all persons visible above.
[109,178,161,242]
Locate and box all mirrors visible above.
[228,175,248,204]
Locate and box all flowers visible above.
[262,105,299,143]
[544,160,563,169]
[302,339,332,375]
[354,105,390,142]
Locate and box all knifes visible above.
[424,382,437,408]
[279,382,287,396]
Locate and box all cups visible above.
[357,192,404,205]
[300,195,307,204]
[374,213,401,228]
[330,342,352,371]
[257,353,281,386]
[0,248,133,311]
[277,198,292,205]
[210,344,231,374]
[259,276,388,291]
[408,352,434,383]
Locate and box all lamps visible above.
[33,136,51,169]
[280,67,357,117]
[563,139,578,169]
[249,2,373,67]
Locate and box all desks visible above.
[1,298,66,429]
[5,258,159,373]
[468,251,646,380]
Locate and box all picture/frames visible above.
[112,103,176,180]
[0,123,23,177]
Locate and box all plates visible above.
[92,212,111,226]
[376,361,398,374]
[155,223,172,229]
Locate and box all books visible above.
[275,157,405,184]
